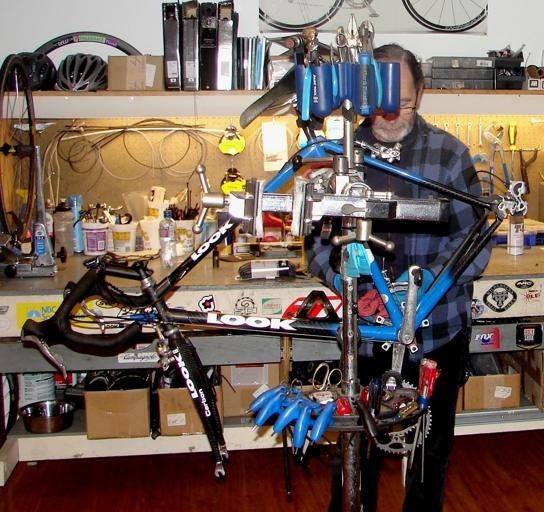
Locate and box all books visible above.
[233,11,266,90]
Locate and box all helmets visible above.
[375,88,421,118]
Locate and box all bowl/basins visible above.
[17,400,75,434]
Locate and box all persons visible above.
[304,43,493,512]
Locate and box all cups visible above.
[111,225,137,253]
[138,220,158,252]
[82,222,110,255]
[148,185,166,220]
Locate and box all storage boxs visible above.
[107,55,166,91]
[82,370,152,440]
[220,362,280,419]
[454,386,463,413]
[153,384,223,438]
[462,353,522,410]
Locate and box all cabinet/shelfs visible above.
[0,88,544,490]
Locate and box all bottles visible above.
[212,247,219,269]
[53,202,74,257]
[507,212,526,255]
[67,193,83,253]
[159,210,178,265]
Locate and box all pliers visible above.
[483,125,510,193]
[346,14,383,108]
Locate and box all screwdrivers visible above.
[507,121,517,169]
[408,358,437,483]
[366,377,382,459]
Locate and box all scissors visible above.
[312,363,343,392]
[280,378,302,393]
